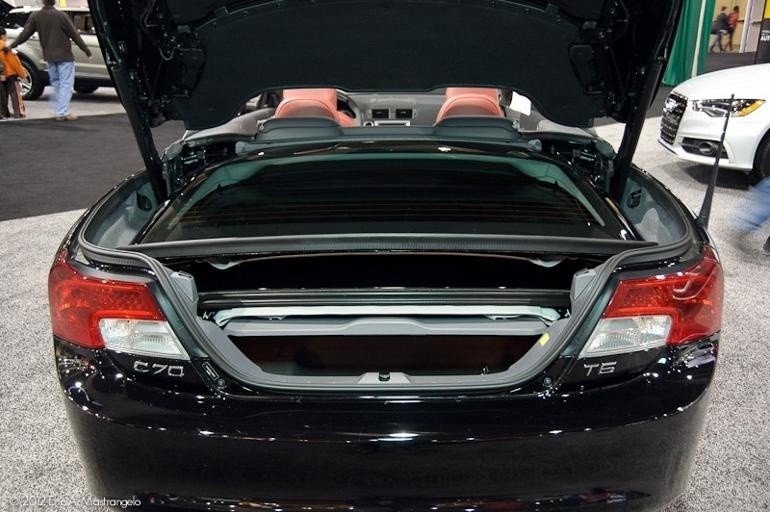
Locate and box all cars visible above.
[0,0,770,512]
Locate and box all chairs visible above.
[56,113,77,121]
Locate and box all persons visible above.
[709,6,728,53]
[722,5,740,52]
[0,0,93,122]
